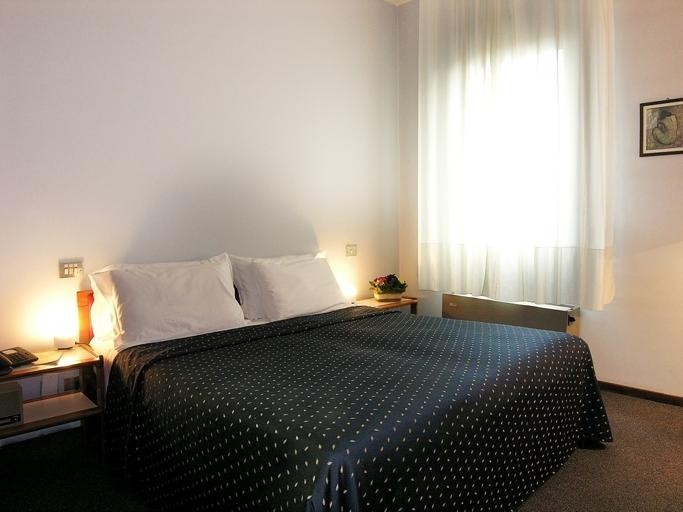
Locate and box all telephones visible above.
[0,346,39,366]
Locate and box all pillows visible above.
[89,251,350,351]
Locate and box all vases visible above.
[373,289,403,302]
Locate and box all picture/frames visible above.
[639,97,683,157]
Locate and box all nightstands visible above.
[1,345,105,469]
[352,296,419,314]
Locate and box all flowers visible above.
[368,273,409,292]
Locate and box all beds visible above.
[75,291,611,511]
